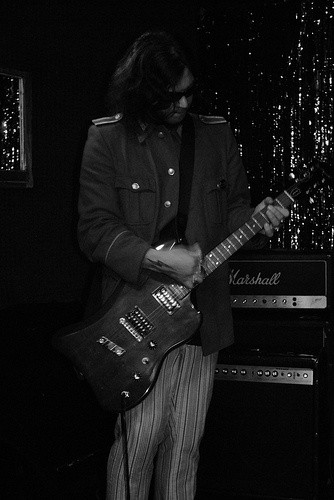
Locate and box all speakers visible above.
[196,310,334,500]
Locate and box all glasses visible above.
[158,78,199,103]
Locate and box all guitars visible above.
[45,156,334,411]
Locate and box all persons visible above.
[76,30,290,500]
